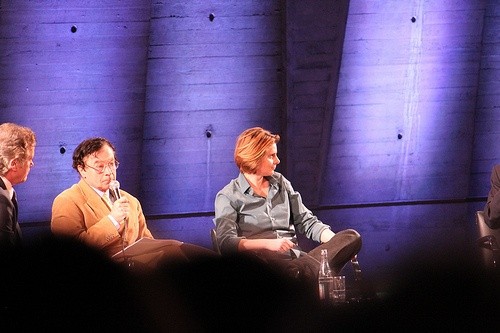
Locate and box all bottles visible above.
[317,249,333,302]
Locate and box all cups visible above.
[332,276,346,304]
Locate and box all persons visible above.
[214,127,363,279]
[50,138,189,273]
[484,162,500,232]
[0,122,38,235]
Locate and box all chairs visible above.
[210,229,219,255]
[477,210,500,281]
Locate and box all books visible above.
[112,237,218,264]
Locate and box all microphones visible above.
[110,180,127,220]
